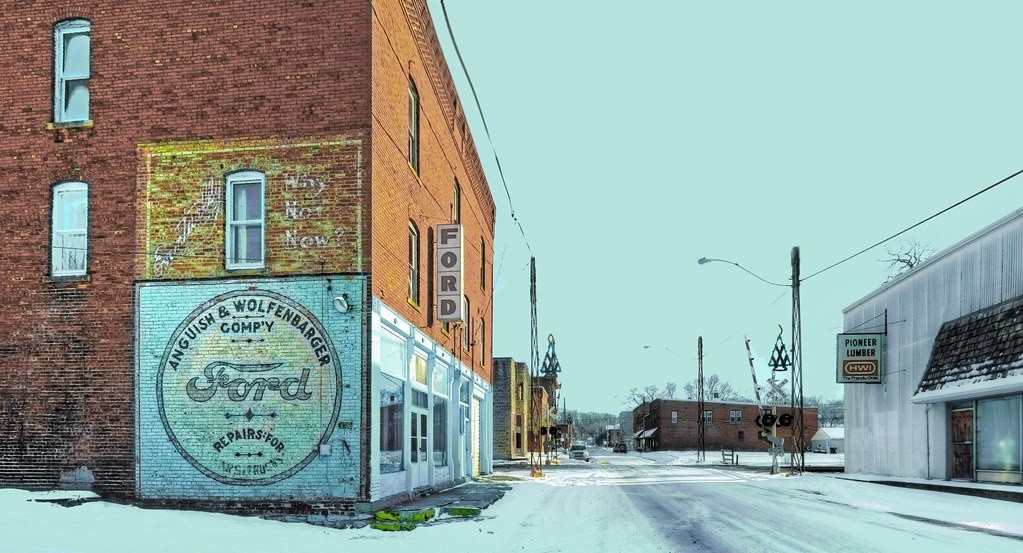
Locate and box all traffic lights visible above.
[762,414,776,426]
[779,413,792,426]
[550,427,557,436]
[541,427,547,435]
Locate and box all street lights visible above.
[696,247,805,473]
[643,345,706,462]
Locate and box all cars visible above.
[613,443,627,453]
[567,443,589,462]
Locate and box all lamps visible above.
[333,293,353,314]
